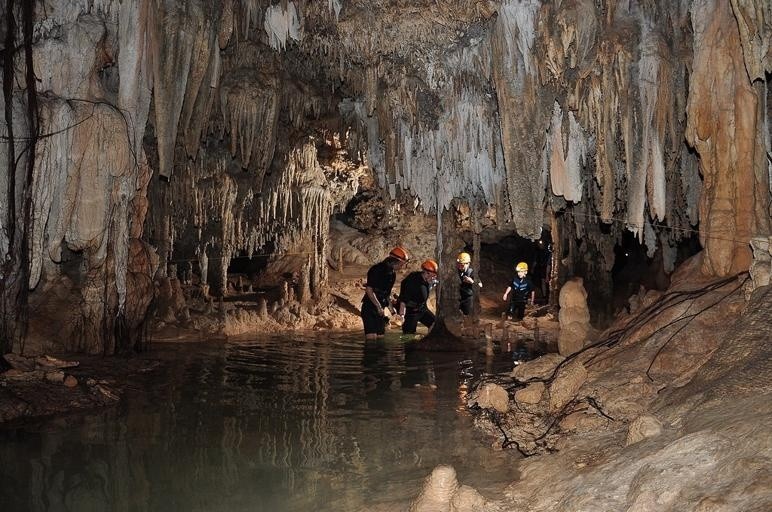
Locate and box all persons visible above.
[502,262,535,320]
[361,247,438,340]
[457,253,483,317]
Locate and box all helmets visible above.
[515,262,529,272]
[421,260,439,273]
[389,248,410,264]
[457,252,472,264]
